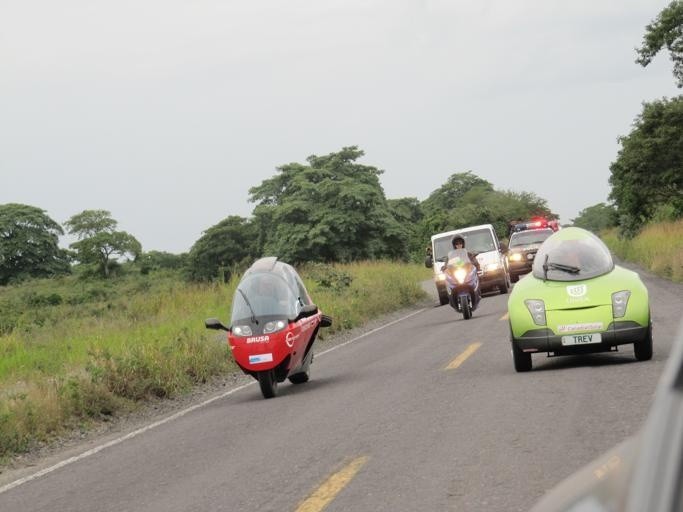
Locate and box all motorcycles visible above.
[436,248,483,319]
[204,257,333,398]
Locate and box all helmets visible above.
[453,235,464,248]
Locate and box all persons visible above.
[440,234,481,275]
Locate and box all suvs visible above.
[505,220,555,283]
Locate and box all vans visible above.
[426,223,510,306]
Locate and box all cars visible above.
[507,227,654,372]
